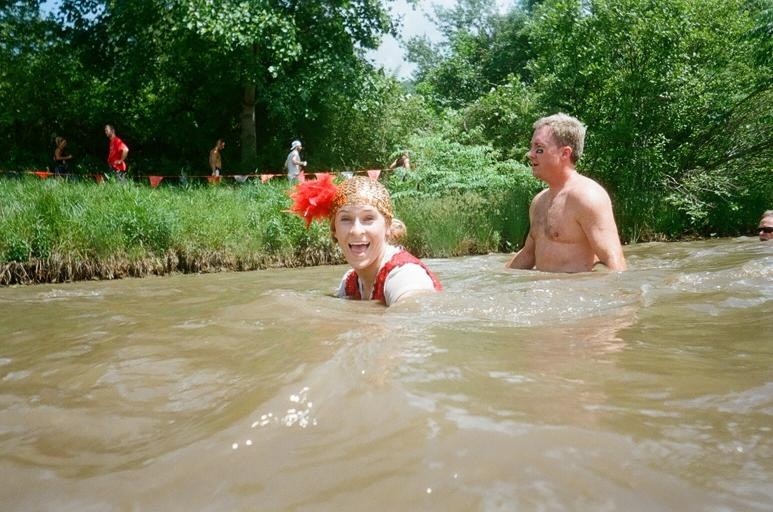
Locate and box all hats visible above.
[290,141,301,150]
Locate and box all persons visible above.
[50,136,76,178]
[283,140,307,181]
[103,123,129,179]
[206,137,224,176]
[506,113,629,275]
[324,175,444,308]
[757,210,772,241]
[389,153,411,170]
